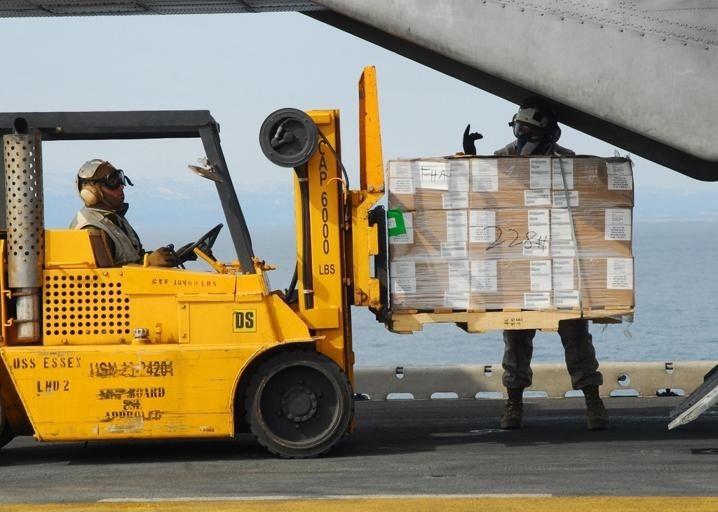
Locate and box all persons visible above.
[462,106,610,431]
[67,158,184,271]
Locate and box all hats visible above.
[512,106,552,130]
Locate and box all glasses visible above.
[103,168,127,189]
[512,121,546,144]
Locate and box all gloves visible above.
[143,246,177,268]
[463,123,483,156]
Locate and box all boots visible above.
[499,387,524,431]
[582,385,611,431]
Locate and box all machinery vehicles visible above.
[0,56,639,456]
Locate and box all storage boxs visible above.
[387,158,635,312]
